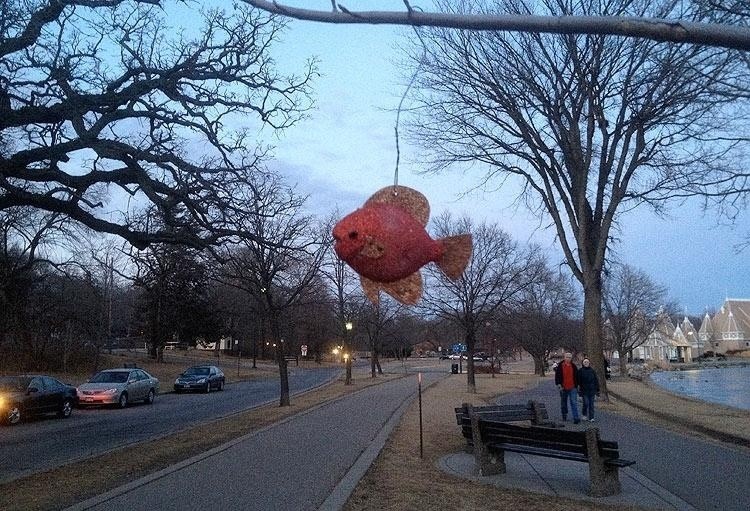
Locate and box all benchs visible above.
[454,400,637,498]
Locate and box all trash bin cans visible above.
[452,364,458,374]
[610,357,621,377]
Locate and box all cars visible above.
[0,368,160,426]
[440,353,484,362]
[174,366,225,394]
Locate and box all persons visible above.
[544,360,559,374]
[554,352,581,426]
[576,356,601,424]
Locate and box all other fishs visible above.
[331,185,475,309]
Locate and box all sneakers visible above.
[573,416,596,424]
